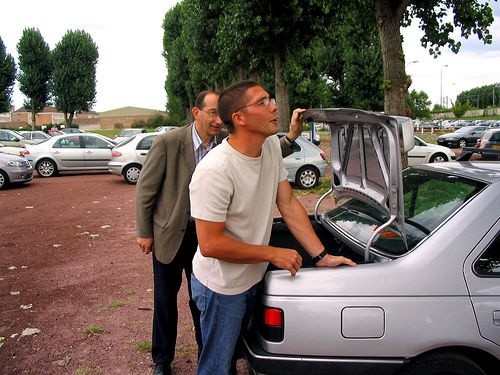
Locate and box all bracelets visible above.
[311,249,327,264]
[286,135,295,143]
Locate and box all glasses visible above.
[198,108,219,117]
[232,97,276,114]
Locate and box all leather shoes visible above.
[151,362,172,375]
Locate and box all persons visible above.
[135,91,306,375]
[189,80,356,375]
[51,124,57,130]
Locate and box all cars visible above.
[244,108,500,375]
[277,131,328,189]
[408,119,500,166]
[0,126,180,190]
